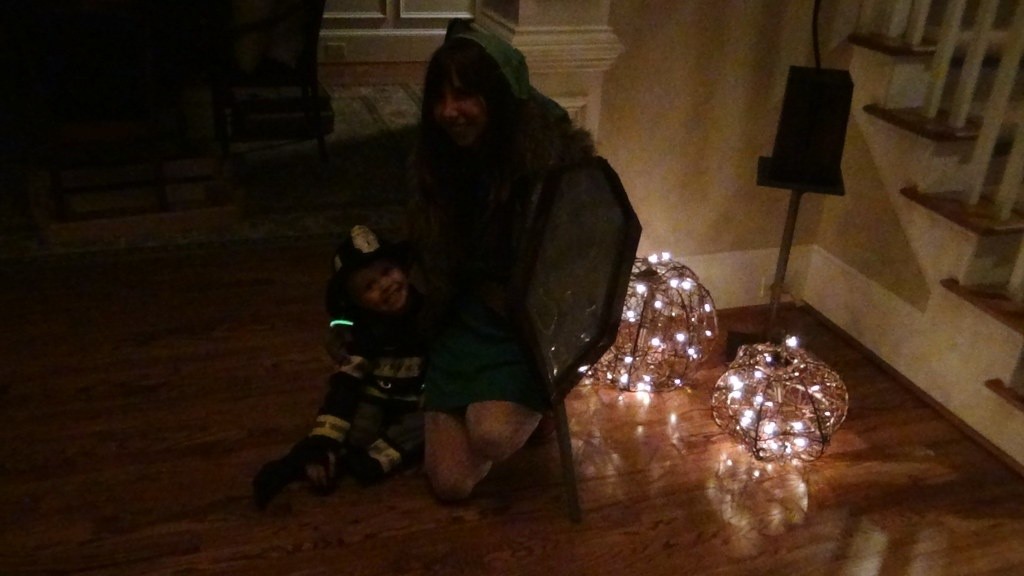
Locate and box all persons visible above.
[242,224,437,507]
[394,19,596,503]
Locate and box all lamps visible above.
[583,257,719,395]
[710,333,846,467]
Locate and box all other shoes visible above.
[531,409,557,438]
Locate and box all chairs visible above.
[7,2,182,223]
[204,0,340,180]
